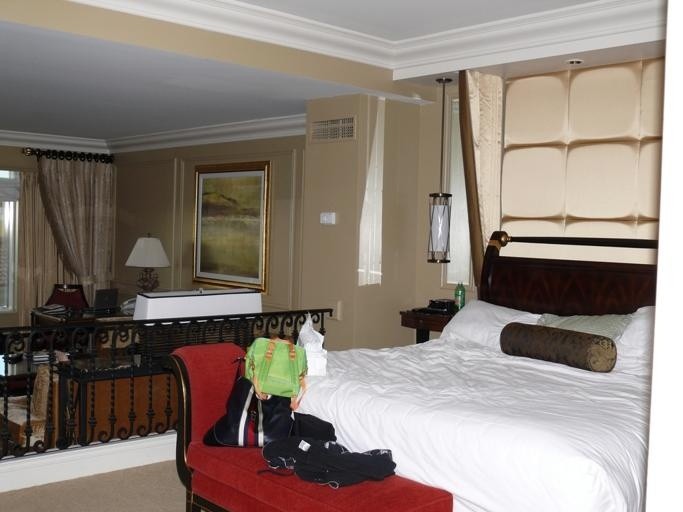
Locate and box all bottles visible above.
[455,281,465,310]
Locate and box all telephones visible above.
[120,297,136,310]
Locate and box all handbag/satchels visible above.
[204,337,397,486]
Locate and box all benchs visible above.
[165,343,453,512]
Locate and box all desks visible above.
[399,310,453,344]
[30,308,133,344]
[0,352,47,394]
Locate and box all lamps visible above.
[124,233,172,293]
[427,78,453,264]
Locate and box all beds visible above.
[293,232,657,512]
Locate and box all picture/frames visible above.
[192,159,271,295]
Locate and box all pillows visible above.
[440,299,654,372]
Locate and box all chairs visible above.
[47,284,89,308]
[0,364,61,448]
